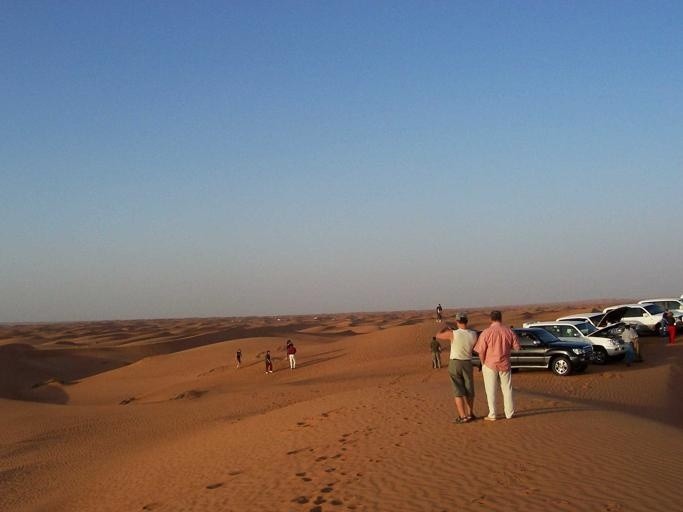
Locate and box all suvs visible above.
[602,298,682,336]
[471,307,630,375]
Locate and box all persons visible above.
[285,339,292,362]
[662,309,669,336]
[435,311,478,425]
[629,323,642,363]
[605,320,611,327]
[430,337,443,369]
[287,343,297,369]
[666,311,677,344]
[234,348,242,369]
[263,349,273,374]
[434,303,442,324]
[621,324,636,368]
[473,310,520,423]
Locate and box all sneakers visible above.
[468,415,475,419]
[453,416,468,423]
[484,417,497,421]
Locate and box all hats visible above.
[456,312,467,321]
